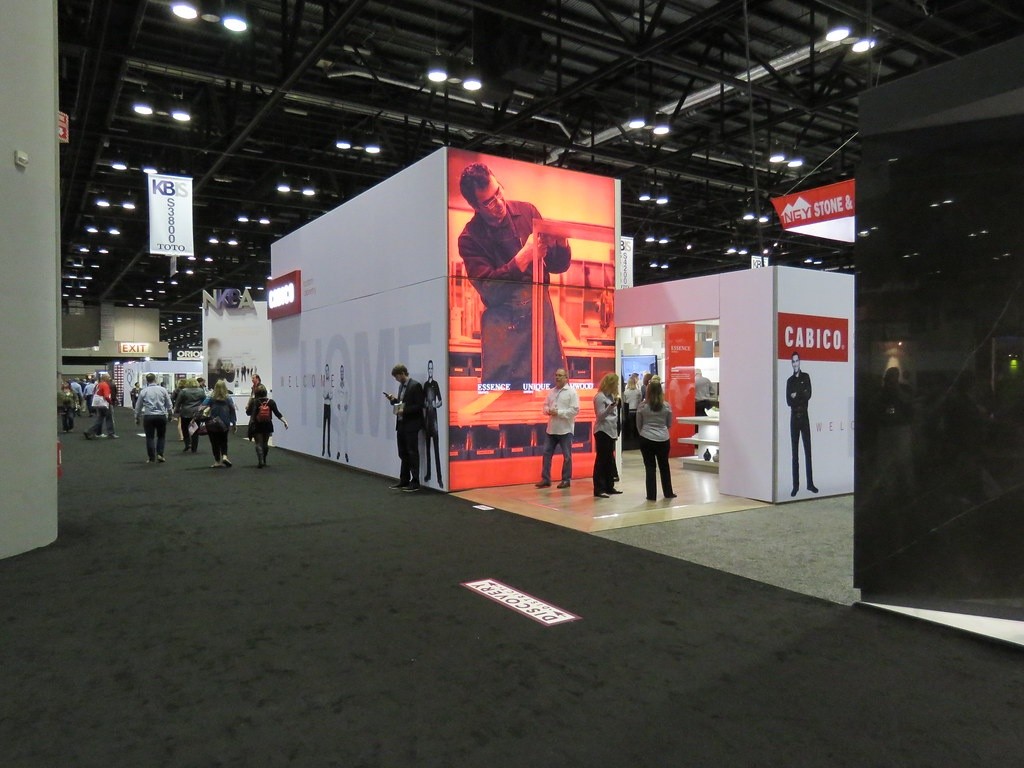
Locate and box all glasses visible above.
[477,181,504,210]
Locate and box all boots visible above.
[255,445,269,469]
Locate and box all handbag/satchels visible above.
[197,421,208,436]
[196,405,212,421]
[90,393,109,410]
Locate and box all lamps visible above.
[77,0,878,276]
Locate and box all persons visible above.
[57,374,119,439]
[874,368,913,501]
[534,369,580,488]
[623,373,660,443]
[456,162,563,386]
[787,352,818,496]
[321,364,349,462]
[422,360,443,488]
[636,381,677,500]
[208,338,253,390]
[202,381,237,467]
[161,378,208,452]
[131,382,143,424]
[135,374,172,462]
[246,374,288,468]
[593,374,624,498]
[695,370,715,448]
[386,365,424,491]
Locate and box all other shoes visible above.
[594,492,610,498]
[146,444,232,468]
[646,496,656,500]
[423,474,432,482]
[328,451,331,458]
[336,451,341,459]
[322,450,326,456]
[666,493,677,498]
[438,479,443,488]
[807,485,819,493]
[534,481,550,487]
[605,488,623,494]
[389,483,410,490]
[84,431,121,440]
[791,487,799,496]
[401,485,420,493]
[345,453,348,462]
[557,480,570,489]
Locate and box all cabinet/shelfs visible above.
[676,415,720,474]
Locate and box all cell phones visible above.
[382,392,390,397]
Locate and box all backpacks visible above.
[255,397,272,423]
